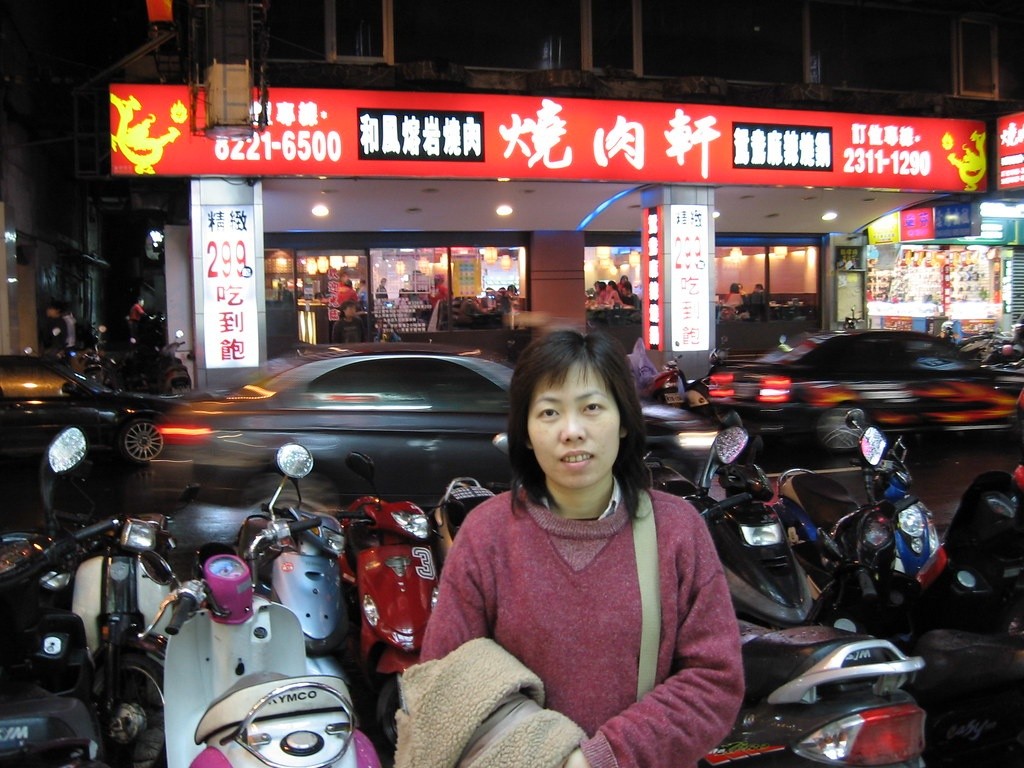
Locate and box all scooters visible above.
[52,329,191,398]
[0,403,1024,768]
[623,336,733,403]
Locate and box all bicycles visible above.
[842,304,872,333]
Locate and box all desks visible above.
[297,302,329,345]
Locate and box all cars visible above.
[960,333,1024,394]
[708,329,1024,457]
[0,354,192,468]
[138,350,729,510]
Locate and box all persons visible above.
[452,285,518,327]
[726,283,770,321]
[129,297,156,339]
[373,270,454,342]
[419,330,745,768]
[585,275,642,324]
[328,272,369,344]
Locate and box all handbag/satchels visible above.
[626,338,658,390]
